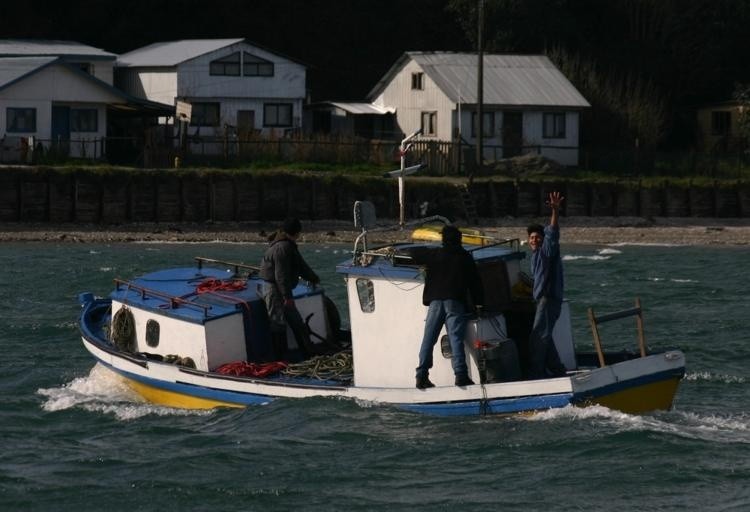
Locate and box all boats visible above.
[77,129,686,421]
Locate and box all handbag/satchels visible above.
[397,245,433,265]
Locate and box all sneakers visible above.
[454,373,475,386]
[415,375,434,389]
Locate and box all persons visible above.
[260,217,321,361]
[416,226,483,386]
[527,190,564,377]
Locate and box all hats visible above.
[283,216,302,233]
